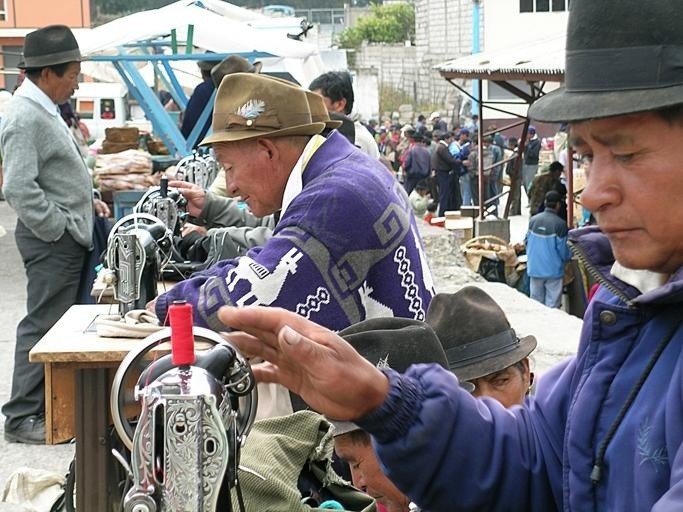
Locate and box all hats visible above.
[198,72,343,145]
[428,286,537,382]
[211,54,262,93]
[417,180,427,188]
[197,50,221,71]
[429,112,440,121]
[545,190,562,201]
[16,25,81,69]
[527,0,680,124]
[327,317,476,440]
[400,125,416,131]
[457,129,469,136]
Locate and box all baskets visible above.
[463,235,513,278]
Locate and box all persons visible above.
[1,25,111,444]
[523,161,574,309]
[425,286,537,410]
[328,317,476,512]
[217,0,683,512]
[308,71,381,161]
[167,112,361,256]
[181,54,262,140]
[361,112,541,217]
[145,72,435,330]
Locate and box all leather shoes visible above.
[4,413,45,444]
